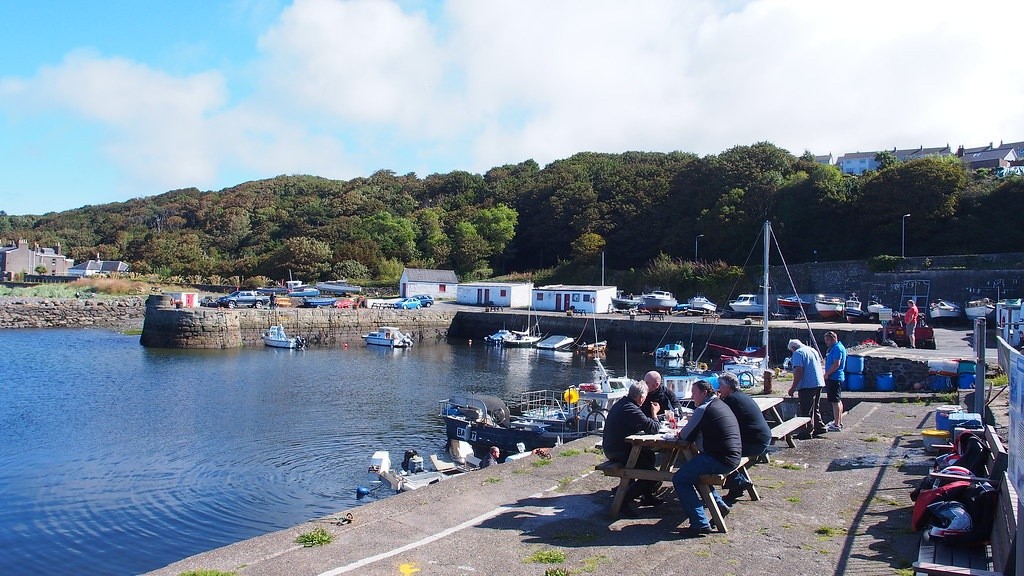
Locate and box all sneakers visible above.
[826,420,843,432]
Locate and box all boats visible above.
[964,301,996,323]
[688,291,718,312]
[655,343,687,358]
[729,295,770,312]
[815,293,906,324]
[361,327,414,347]
[369,220,824,494]
[641,286,680,311]
[261,324,302,348]
[684,360,709,376]
[778,298,811,316]
[610,289,642,312]
[929,299,962,321]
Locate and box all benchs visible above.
[702,455,756,482]
[911,470,1018,576]
[595,448,659,471]
[929,423,1006,487]
[769,416,812,439]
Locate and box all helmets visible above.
[932,464,978,499]
[933,453,962,473]
[927,501,973,543]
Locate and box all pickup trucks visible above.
[218,291,269,309]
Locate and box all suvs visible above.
[412,294,435,308]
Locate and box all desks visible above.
[701,314,718,323]
[607,417,761,533]
[371,303,399,309]
[488,305,503,312]
[745,316,763,325]
[751,397,797,463]
[648,313,664,321]
[573,309,586,317]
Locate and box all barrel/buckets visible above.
[928,360,976,391]
[935,405,962,438]
[841,354,865,391]
[875,372,895,391]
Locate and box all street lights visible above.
[901,213,911,259]
[695,235,704,263]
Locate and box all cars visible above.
[878,312,934,347]
[395,297,422,309]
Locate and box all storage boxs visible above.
[930,374,958,392]
[948,412,983,442]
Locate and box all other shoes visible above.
[740,480,749,490]
[722,486,742,501]
[817,426,828,433]
[637,482,663,503]
[620,502,640,517]
[710,503,730,526]
[793,432,811,439]
[679,525,711,534]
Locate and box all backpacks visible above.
[944,430,988,477]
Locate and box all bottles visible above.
[663,407,680,429]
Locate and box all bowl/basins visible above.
[921,430,950,452]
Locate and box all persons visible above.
[643,370,682,421]
[824,331,846,431]
[788,338,827,440]
[269,291,276,308]
[603,380,660,516]
[630,292,633,300]
[718,372,772,502]
[479,446,500,468]
[617,290,620,298]
[673,380,742,535]
[905,300,918,349]
[1018,331,1024,351]
